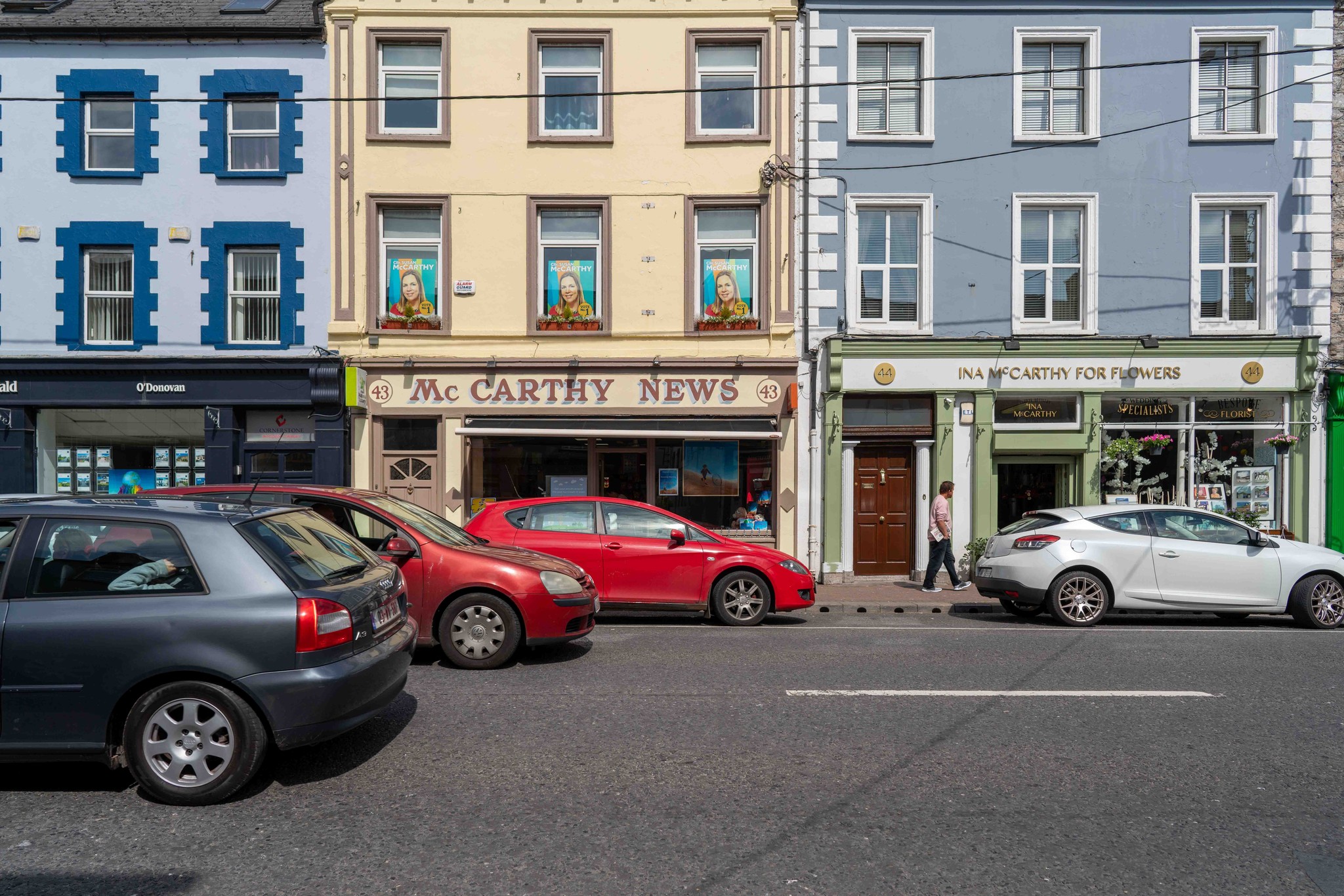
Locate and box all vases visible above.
[697,319,758,331]
[381,320,408,329]
[412,321,440,330]
[1275,442,1290,455]
[538,320,600,330]
[1149,444,1162,455]
[1120,449,1130,454]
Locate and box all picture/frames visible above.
[1105,494,1138,505]
[1195,482,1228,515]
[1230,465,1275,521]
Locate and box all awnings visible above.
[455,419,783,440]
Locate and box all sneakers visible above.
[922,587,942,593]
[954,581,971,590]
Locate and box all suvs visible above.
[0,489,422,808]
[90,481,599,669]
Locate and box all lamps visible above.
[1135,334,1159,348]
[999,335,1020,350]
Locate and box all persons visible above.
[548,271,595,320]
[700,464,712,485]
[107,548,197,591]
[50,530,98,564]
[388,269,435,317]
[705,270,750,319]
[922,481,972,592]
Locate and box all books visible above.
[757,491,772,506]
[762,467,771,480]
[747,502,758,514]
[739,519,768,530]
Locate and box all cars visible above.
[458,495,818,628]
[973,505,1344,631]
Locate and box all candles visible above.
[1196,437,1213,458]
[1139,485,1186,506]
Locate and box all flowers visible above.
[376,312,409,325]
[1103,437,1143,460]
[1215,508,1260,529]
[408,315,442,327]
[1263,434,1299,447]
[537,302,604,327]
[698,301,761,324]
[1140,434,1173,449]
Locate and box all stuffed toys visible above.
[751,514,765,530]
[732,507,747,530]
